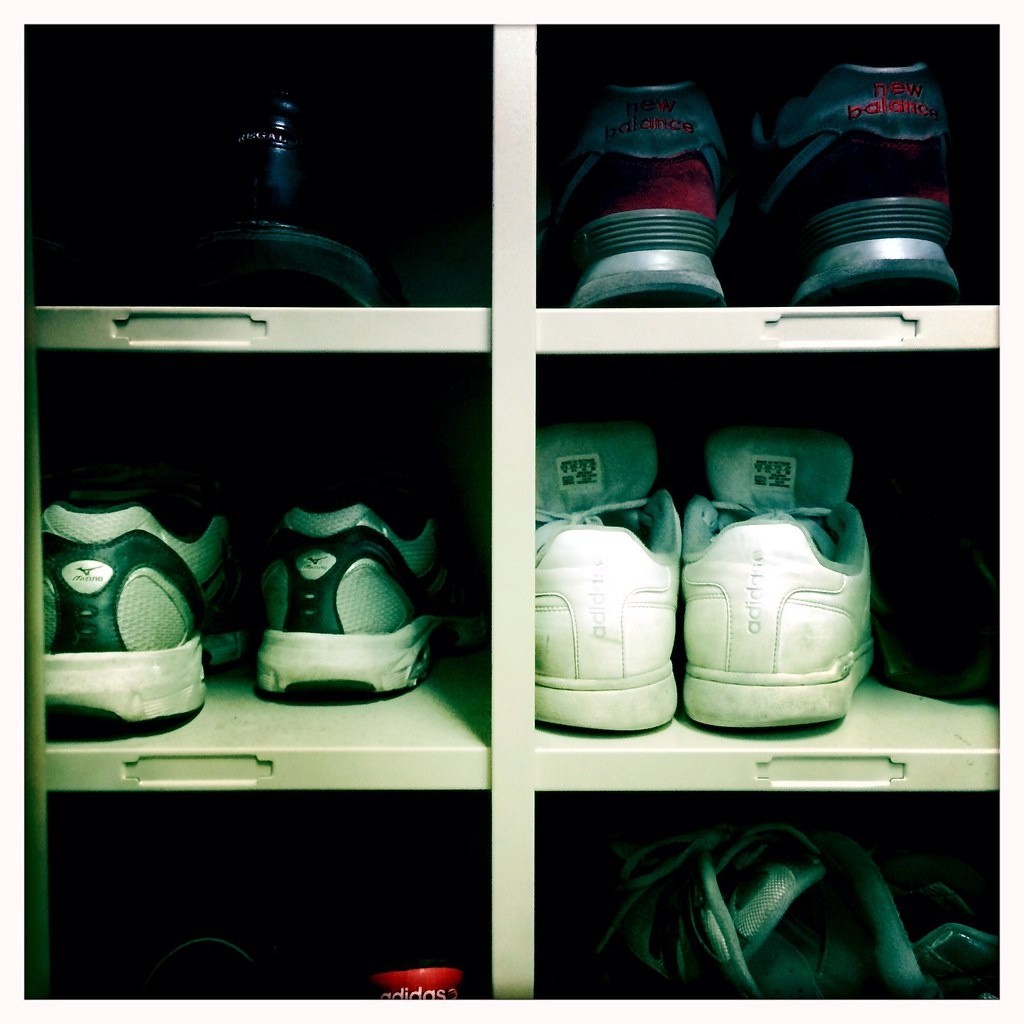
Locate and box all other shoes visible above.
[586,823,1000,999]
[133,909,482,1000]
[189,82,393,305]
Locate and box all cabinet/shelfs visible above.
[24,23,999,999]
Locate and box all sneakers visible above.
[257,505,490,697]
[728,63,963,305]
[40,500,257,724]
[549,80,729,307]
[532,422,681,732]
[679,425,874,729]
